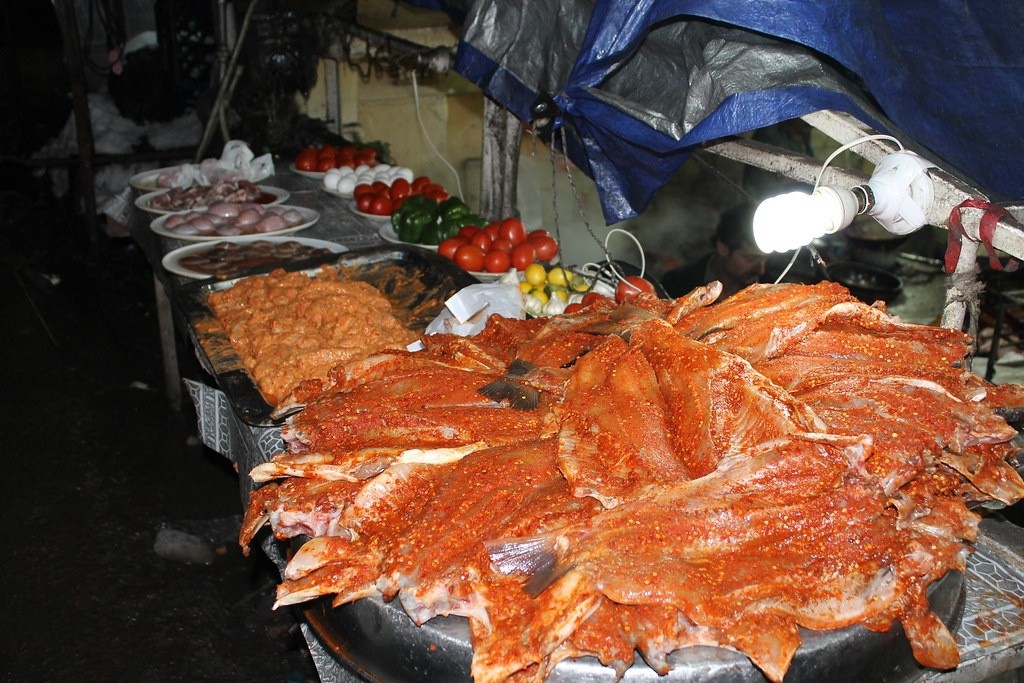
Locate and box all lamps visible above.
[751,151,937,254]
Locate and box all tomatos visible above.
[438,216,655,314]
[354,176,449,215]
[296,143,376,171]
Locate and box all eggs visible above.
[323,164,414,193]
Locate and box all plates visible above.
[379,216,443,254]
[319,181,354,198]
[518,272,615,319]
[162,236,348,280]
[181,244,533,427]
[288,159,381,179]
[435,250,561,284]
[129,164,269,189]
[149,204,319,241]
[134,184,290,214]
[348,188,450,222]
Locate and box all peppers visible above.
[391,194,490,246]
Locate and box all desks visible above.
[123,154,611,475]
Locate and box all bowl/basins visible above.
[822,263,902,305]
[847,218,910,250]
